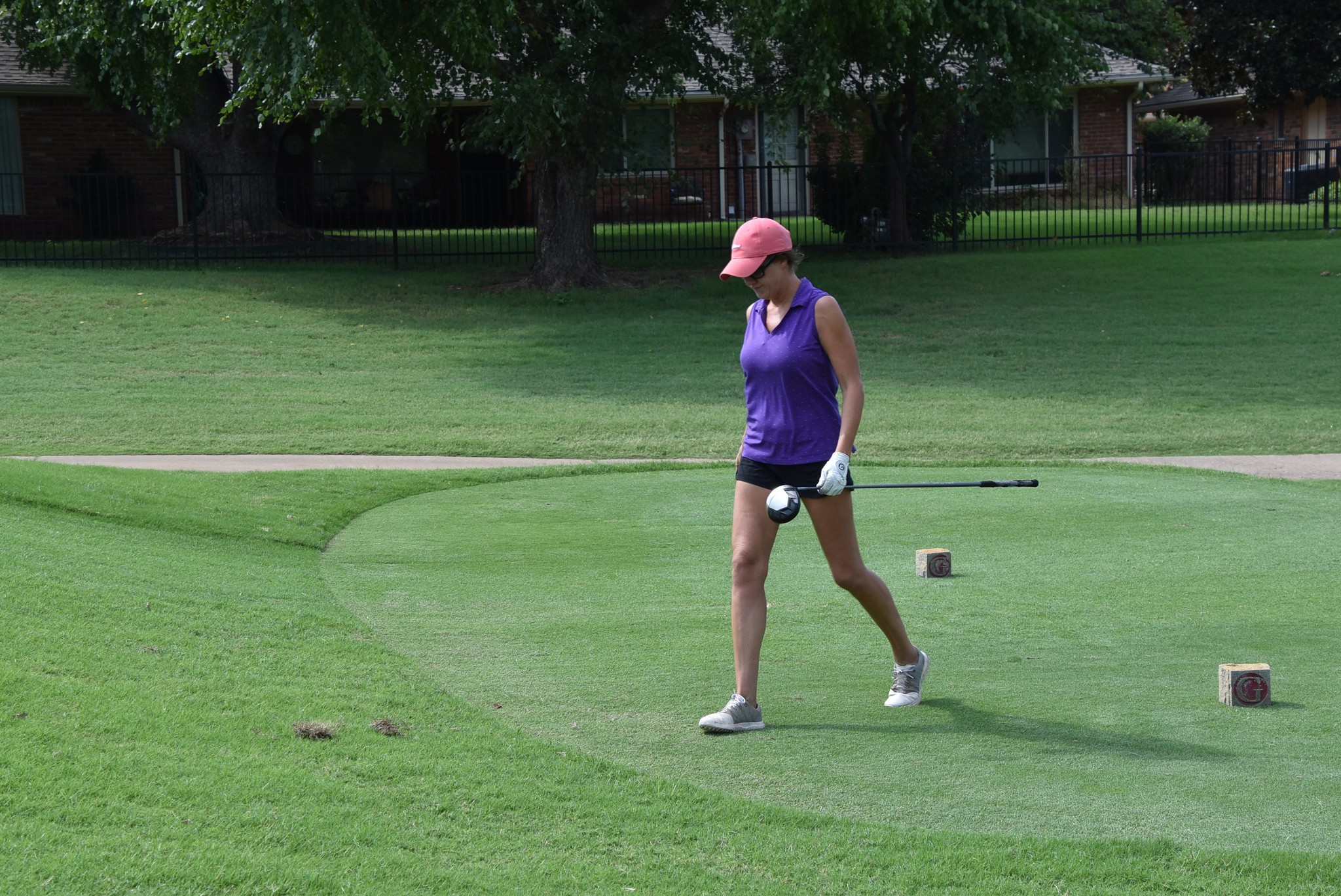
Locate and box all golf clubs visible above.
[764,476,1041,525]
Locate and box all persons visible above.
[698,217,929,734]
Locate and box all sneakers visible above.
[885,644,927,708]
[698,691,765,732]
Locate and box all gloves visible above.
[815,452,850,497]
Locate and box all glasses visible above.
[742,253,780,280]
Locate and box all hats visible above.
[719,216,793,282]
